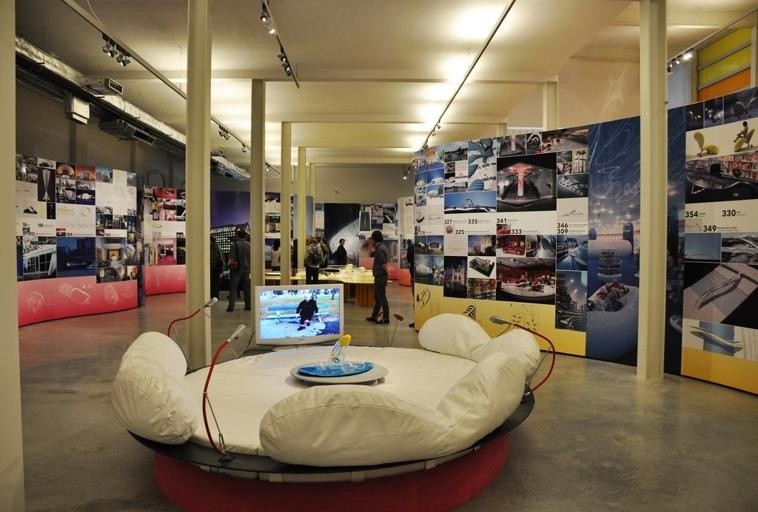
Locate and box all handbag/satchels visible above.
[227,258,240,271]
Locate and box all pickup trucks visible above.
[503,247,525,255]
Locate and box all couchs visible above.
[109,312,556,512]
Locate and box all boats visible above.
[266,311,330,322]
[500,274,555,297]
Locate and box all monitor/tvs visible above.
[254,283,344,352]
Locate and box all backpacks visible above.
[310,245,323,266]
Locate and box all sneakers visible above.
[244,307,250,310]
[367,317,377,321]
[227,308,232,311]
[378,319,390,324]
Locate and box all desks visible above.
[248,269,394,307]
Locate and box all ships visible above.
[597,249,622,282]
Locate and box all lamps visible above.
[277,51,293,79]
[98,38,133,72]
[219,128,230,142]
[238,146,247,155]
[259,12,279,38]
[665,48,694,78]
[399,122,448,179]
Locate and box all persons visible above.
[733,122,749,143]
[335,238,347,266]
[304,236,330,284]
[366,230,390,324]
[265,241,272,268]
[271,240,281,271]
[210,230,250,313]
[296,294,318,331]
